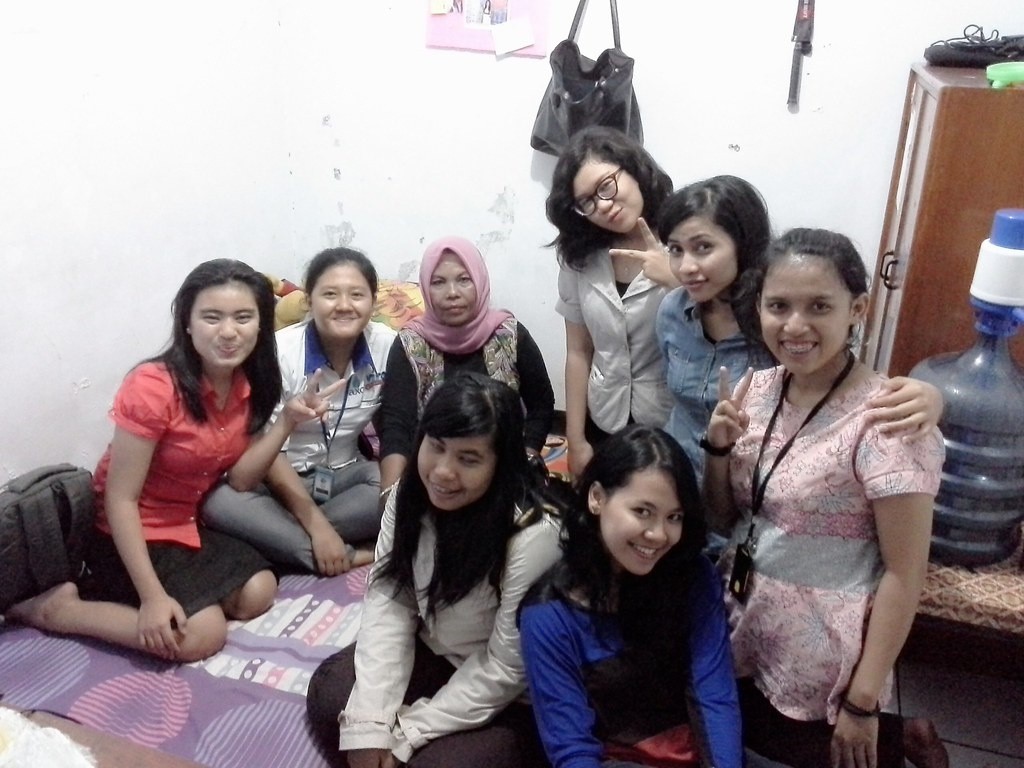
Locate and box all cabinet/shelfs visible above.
[861,62,1023,379]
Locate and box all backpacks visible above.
[1,463,93,612]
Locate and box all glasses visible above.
[571,167,622,216]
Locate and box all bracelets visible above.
[379,485,394,500]
[836,691,882,718]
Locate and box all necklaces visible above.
[620,238,648,273]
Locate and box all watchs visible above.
[700,429,736,457]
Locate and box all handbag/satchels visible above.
[530,0,645,156]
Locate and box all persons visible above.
[3,257,347,664]
[514,422,793,768]
[304,371,564,768]
[539,125,685,494]
[700,231,949,768]
[375,236,557,506]
[197,248,399,578]
[655,174,944,497]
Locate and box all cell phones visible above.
[311,466,335,502]
[728,543,757,604]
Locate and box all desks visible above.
[916,543,1024,652]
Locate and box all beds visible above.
[1,549,377,767]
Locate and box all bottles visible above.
[906,317,1024,567]
